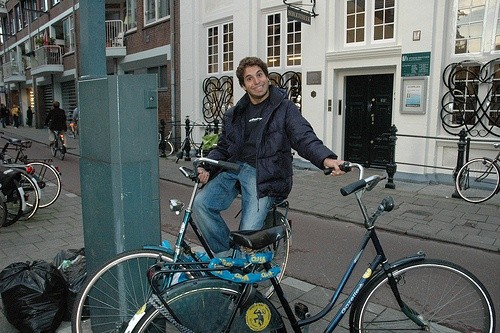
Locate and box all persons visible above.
[73,107,79,135]
[0,103,21,128]
[27,106,35,127]
[190,57,354,258]
[43,101,68,153]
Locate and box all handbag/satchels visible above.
[0,259,66,333]
[52,247,90,321]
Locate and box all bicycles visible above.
[157,118,230,164]
[72,157,293,333]
[118,160,496,333]
[456,142,500,203]
[0,131,62,229]
[44,123,66,160]
